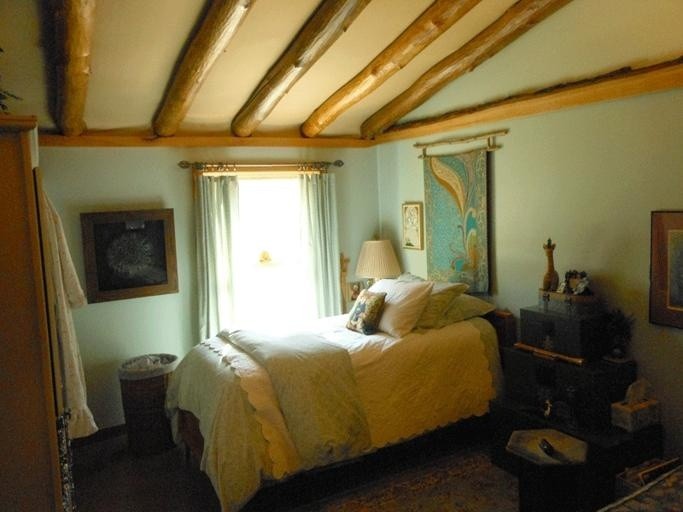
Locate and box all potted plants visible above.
[598,308,635,363]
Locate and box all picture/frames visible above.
[400,202,423,251]
[77,207,179,305]
[648,212,683,329]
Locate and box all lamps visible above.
[355,238,401,286]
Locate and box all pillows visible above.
[344,272,495,337]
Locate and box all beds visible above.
[167,308,515,510]
